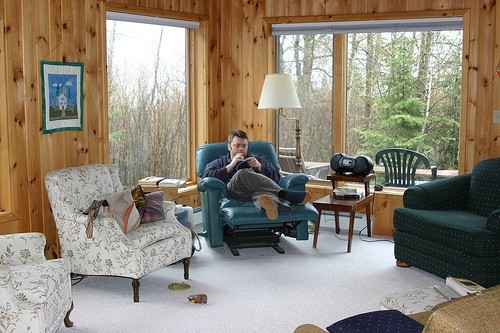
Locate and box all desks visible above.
[313,172,375,253]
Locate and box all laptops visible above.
[332,186,358,199]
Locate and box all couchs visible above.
[294,284,500,333]
[197,141,318,256]
[392,156,500,288]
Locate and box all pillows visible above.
[96,188,142,234]
[142,191,166,222]
[326,310,425,333]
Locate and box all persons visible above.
[204,129,311,220]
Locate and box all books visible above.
[138,176,180,187]
[446,277,487,296]
[434,285,460,299]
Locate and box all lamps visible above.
[258,73,302,172]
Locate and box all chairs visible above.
[277,147,307,174]
[374,149,438,186]
[0,232,75,333]
[43,163,194,304]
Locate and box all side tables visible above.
[371,187,408,235]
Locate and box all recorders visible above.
[329,153,374,176]
